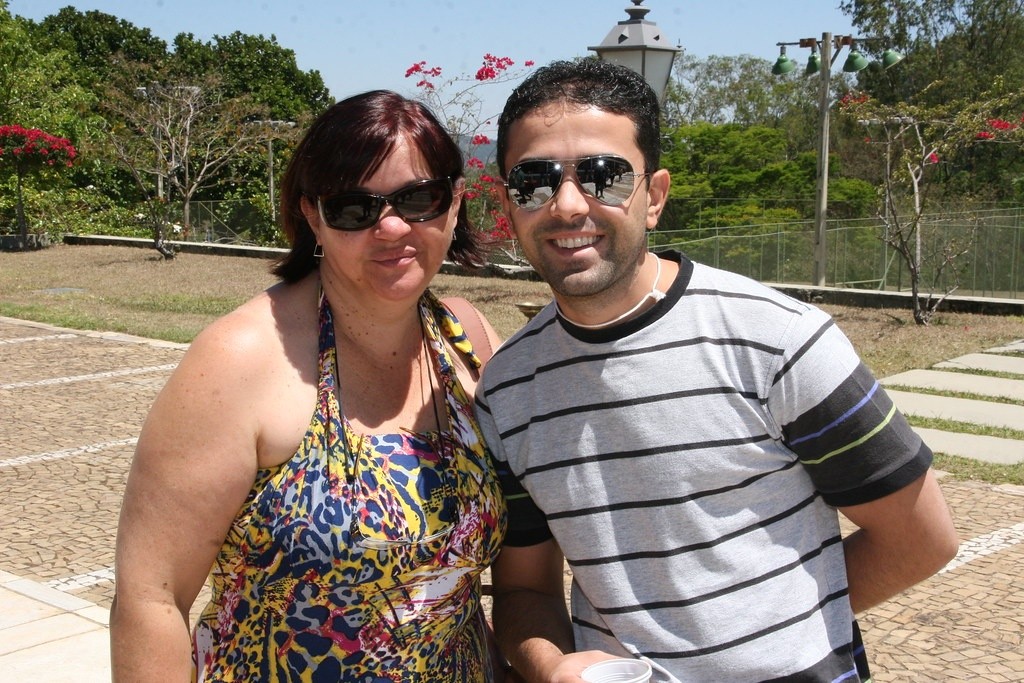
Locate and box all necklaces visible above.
[551,252,666,331]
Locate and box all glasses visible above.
[315,177,453,231]
[503,154,651,212]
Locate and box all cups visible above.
[579,658,652,683]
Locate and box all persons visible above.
[201,220,212,242]
[107,89,504,682]
[474,56,960,683]
[171,221,189,241]
